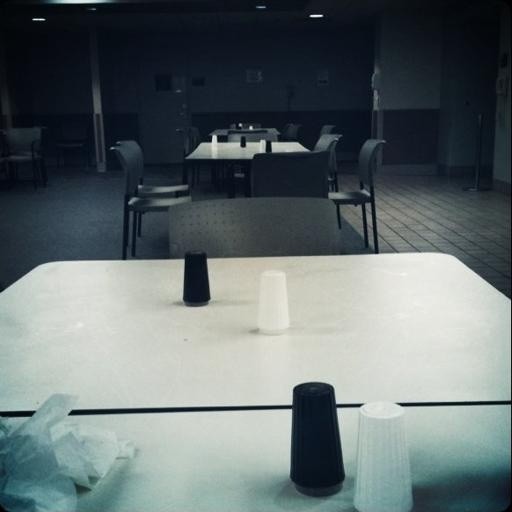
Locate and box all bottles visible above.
[249,126,253,130]
[240,136,246,148]
[289,381,346,497]
[182,248,212,308]
[352,402,414,512]
[212,135,217,144]
[255,270,290,335]
[260,140,272,153]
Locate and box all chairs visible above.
[105,120,389,261]
[1,109,144,194]
